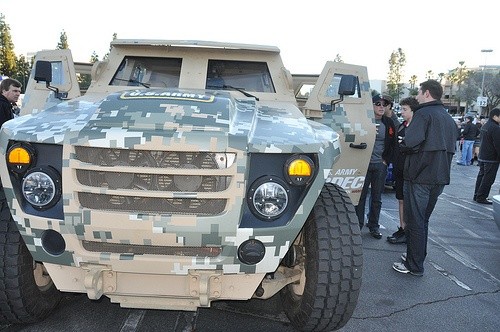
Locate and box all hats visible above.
[373,94,394,103]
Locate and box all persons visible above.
[392,79,458,278]
[0,78,22,130]
[354,90,500,245]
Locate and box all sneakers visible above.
[391,261,423,277]
[401,252,408,260]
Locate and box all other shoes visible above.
[473,197,494,204]
[386,226,406,244]
[370,230,382,239]
[456,161,463,165]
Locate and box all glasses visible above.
[374,103,389,107]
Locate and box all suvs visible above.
[0,36,377,332]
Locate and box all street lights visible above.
[456,60,465,114]
[477,49,493,119]
